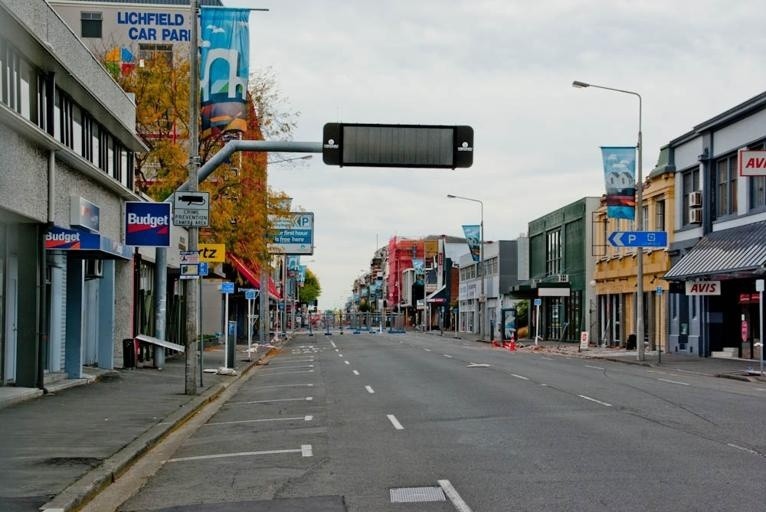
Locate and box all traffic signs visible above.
[266,214,312,253]
[607,231,668,247]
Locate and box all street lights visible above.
[446,193,486,341]
[572,79,644,364]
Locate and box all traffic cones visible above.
[510,330,516,351]
